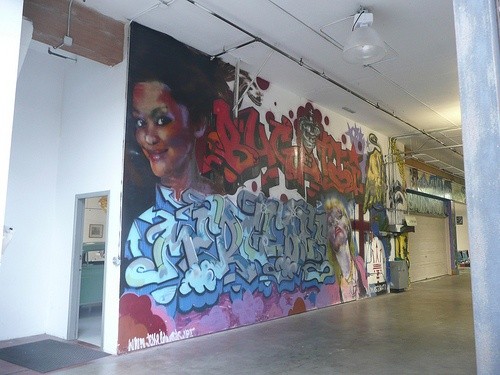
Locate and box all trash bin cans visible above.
[391,261,409,292]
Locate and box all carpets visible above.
[0,339,112,373]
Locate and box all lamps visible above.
[343,6,387,67]
